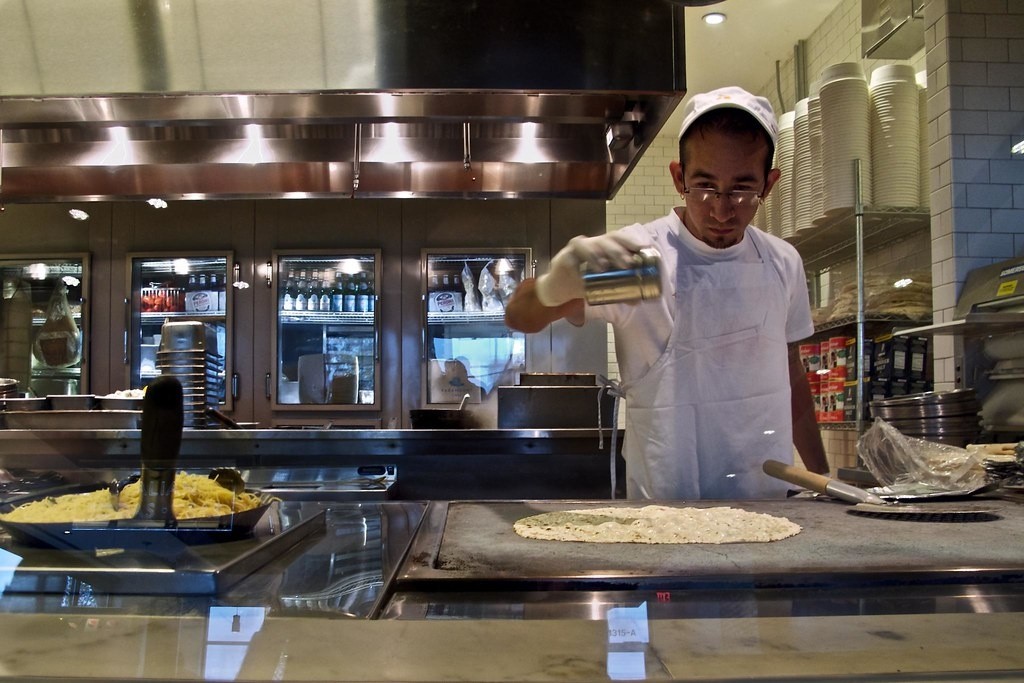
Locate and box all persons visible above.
[505,86,829,500]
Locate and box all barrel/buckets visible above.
[431,358,481,404]
[298,354,359,404]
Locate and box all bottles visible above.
[283,267,374,312]
[428,273,461,291]
[185,272,226,312]
[581,251,663,306]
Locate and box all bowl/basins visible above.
[155,321,208,427]
[746,61,931,239]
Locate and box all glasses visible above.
[679,161,771,208]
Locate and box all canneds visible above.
[797,336,852,423]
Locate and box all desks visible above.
[0,429,626,500]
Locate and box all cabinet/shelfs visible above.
[0,202,609,431]
[787,157,934,487]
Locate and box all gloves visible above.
[534,229,660,308]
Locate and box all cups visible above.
[359,389,374,404]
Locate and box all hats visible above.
[679,86,779,151]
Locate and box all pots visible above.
[0,377,273,548]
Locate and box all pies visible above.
[513,504,803,545]
[812,276,933,325]
